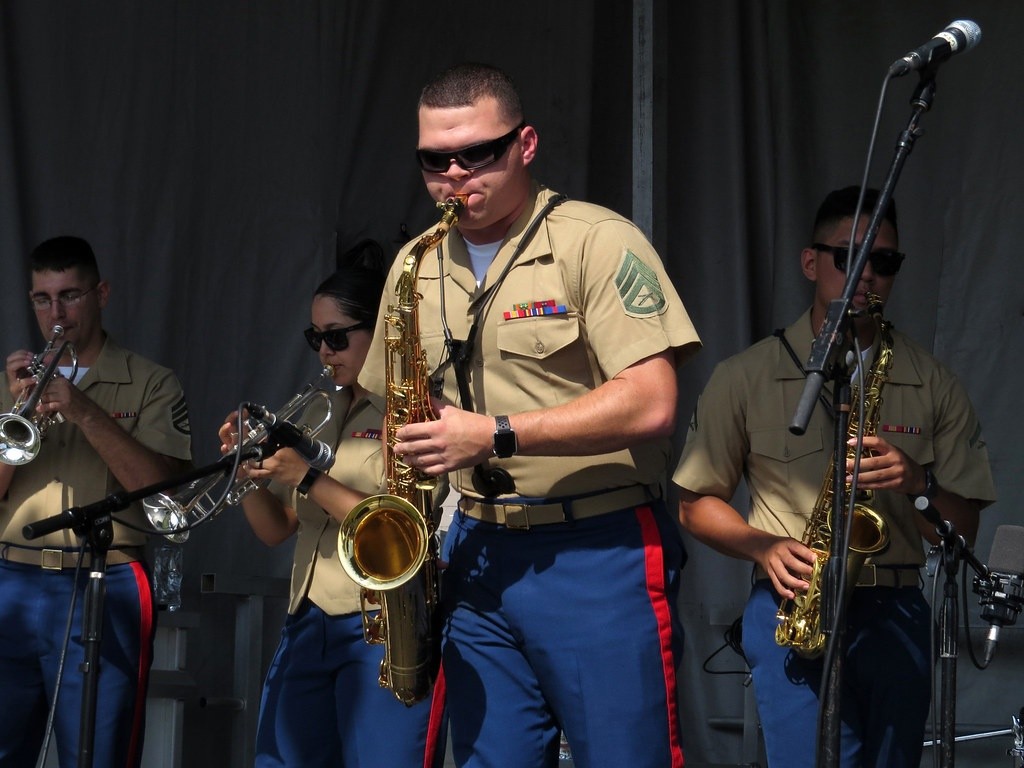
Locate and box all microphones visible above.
[888,19,981,77]
[246,401,336,470]
[984,524,1024,662]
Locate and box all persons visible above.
[0,235,193,768]
[670,184,1000,768]
[357,63,706,768]
[217,262,432,768]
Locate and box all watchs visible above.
[492,413,518,459]
[905,465,938,501]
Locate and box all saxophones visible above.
[336,194,466,706]
[774,290,895,657]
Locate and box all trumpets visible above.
[0,326,79,467]
[142,364,335,544]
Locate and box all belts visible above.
[754,560,920,588]
[0,543,150,571]
[449,482,663,530]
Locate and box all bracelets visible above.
[297,468,319,496]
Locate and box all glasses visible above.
[805,241,905,279]
[413,119,526,173]
[29,282,98,311]
[303,318,377,352]
